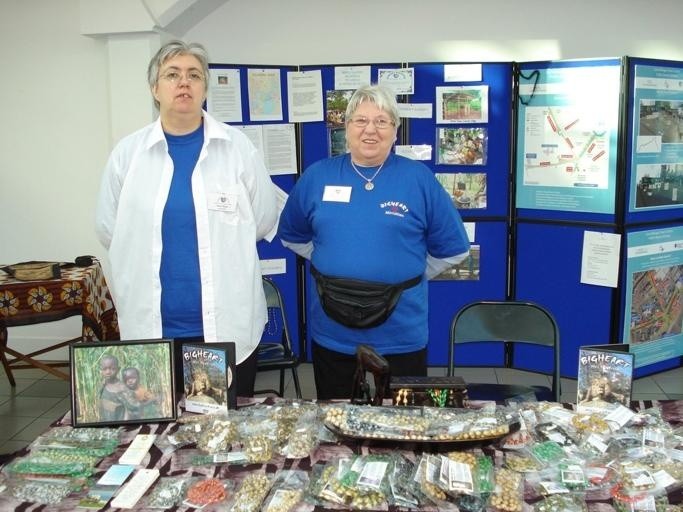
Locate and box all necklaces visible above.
[347,156,385,192]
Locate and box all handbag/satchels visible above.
[306,260,423,332]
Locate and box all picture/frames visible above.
[67,337,178,429]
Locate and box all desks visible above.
[0,258,102,387]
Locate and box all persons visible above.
[93,354,129,421]
[275,82,474,401]
[89,38,279,398]
[118,367,153,419]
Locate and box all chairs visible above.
[448,299,562,404]
[252,276,302,398]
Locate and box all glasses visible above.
[349,115,395,129]
[158,70,208,84]
